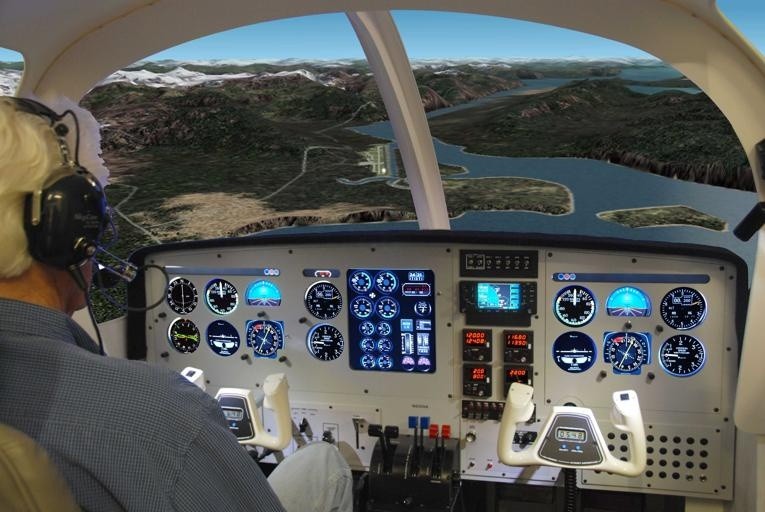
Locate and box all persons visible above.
[0,96,355,512]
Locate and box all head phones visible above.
[8,94,108,272]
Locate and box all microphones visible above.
[72,235,139,285]
